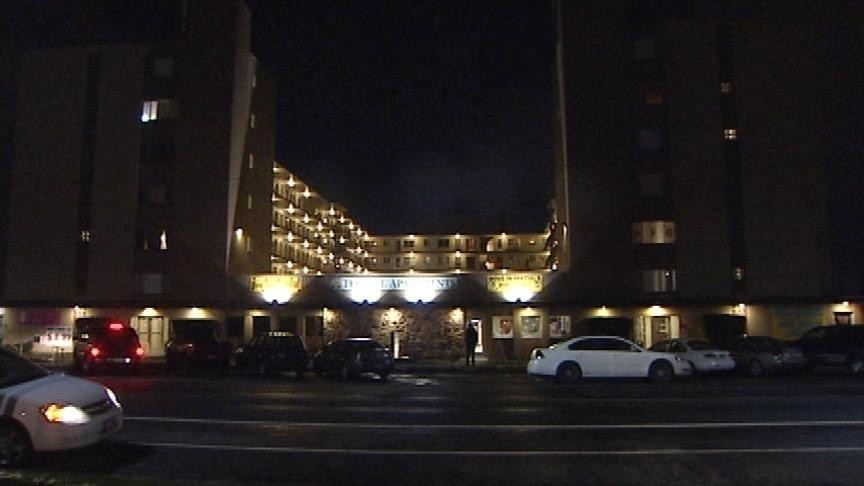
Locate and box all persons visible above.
[466,324,478,366]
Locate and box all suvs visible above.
[234,330,311,376]
[72,322,143,374]
[793,322,864,378]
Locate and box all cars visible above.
[0,339,126,467]
[644,337,738,381]
[309,334,397,382]
[723,332,814,379]
[522,334,696,389]
[162,326,248,368]
